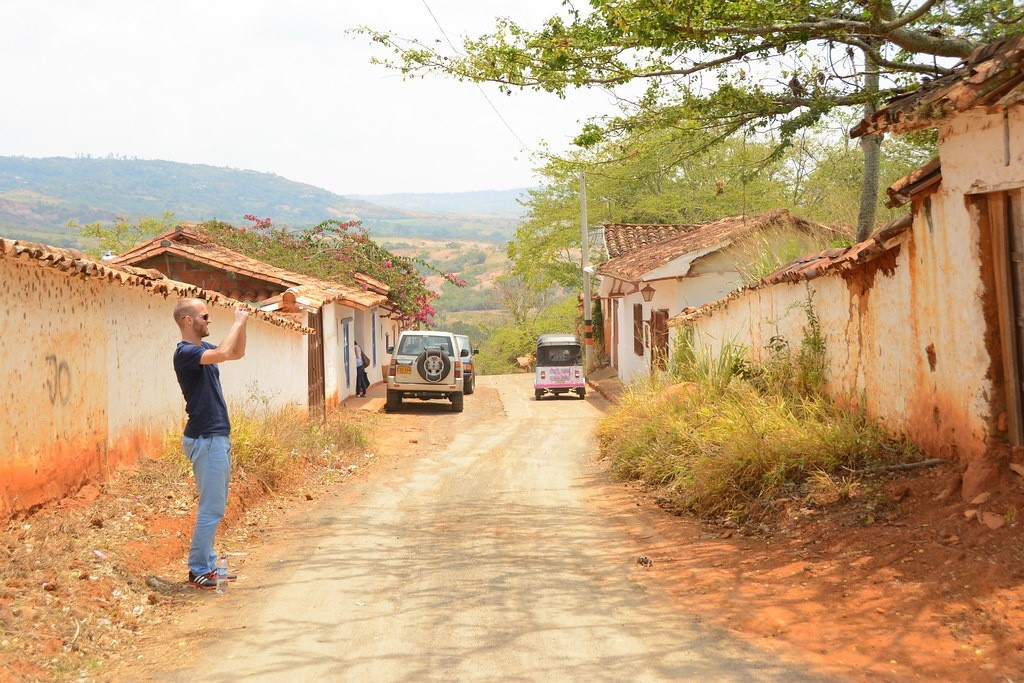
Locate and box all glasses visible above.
[182,313,209,320]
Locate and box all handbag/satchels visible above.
[361,373,369,388]
[361,351,370,368]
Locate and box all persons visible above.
[354,340,366,398]
[173,297,251,590]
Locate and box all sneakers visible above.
[212,568,237,582]
[189,571,217,588]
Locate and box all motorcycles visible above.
[532,333,586,400]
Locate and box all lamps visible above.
[641,281,656,302]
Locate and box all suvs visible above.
[421,334,479,394]
[386,330,469,413]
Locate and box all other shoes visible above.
[357,395,360,398]
[360,394,367,396]
